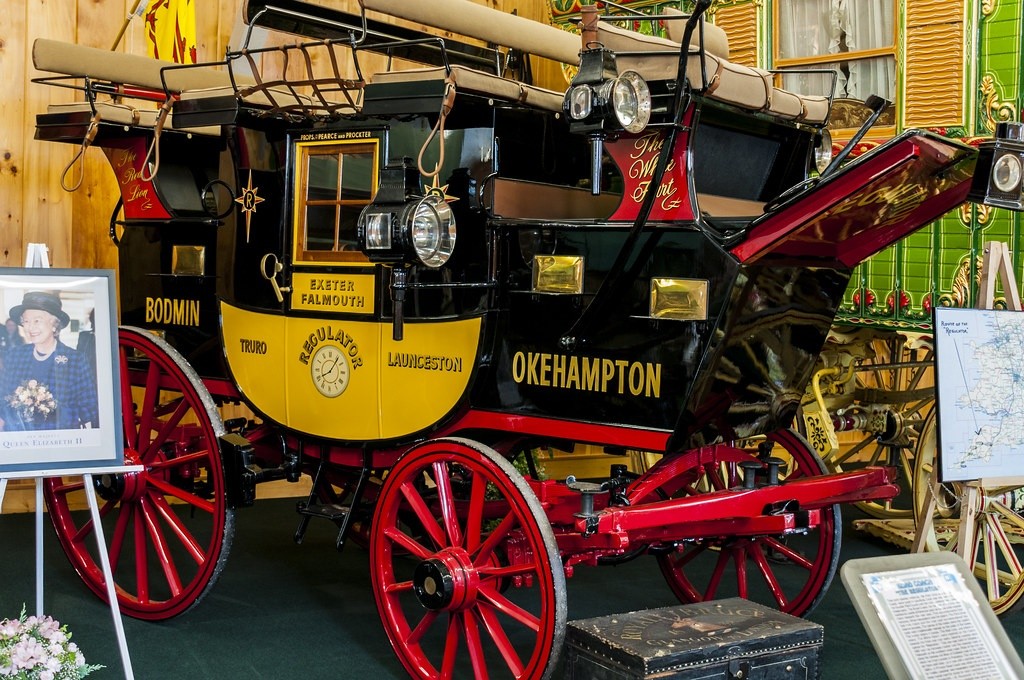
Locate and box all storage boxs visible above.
[564,596,824,680]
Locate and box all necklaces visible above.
[32,341,57,361]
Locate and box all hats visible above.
[9,292,70,329]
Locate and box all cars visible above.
[33,1,995,679]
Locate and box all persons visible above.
[0,292,100,431]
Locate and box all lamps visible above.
[357,154,498,341]
[812,127,833,174]
[563,41,652,197]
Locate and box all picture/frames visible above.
[0,265,125,471]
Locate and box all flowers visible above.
[4,377,60,431]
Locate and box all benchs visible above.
[31,34,294,139]
[568,0,837,127]
[342,0,566,111]
[160,0,505,127]
